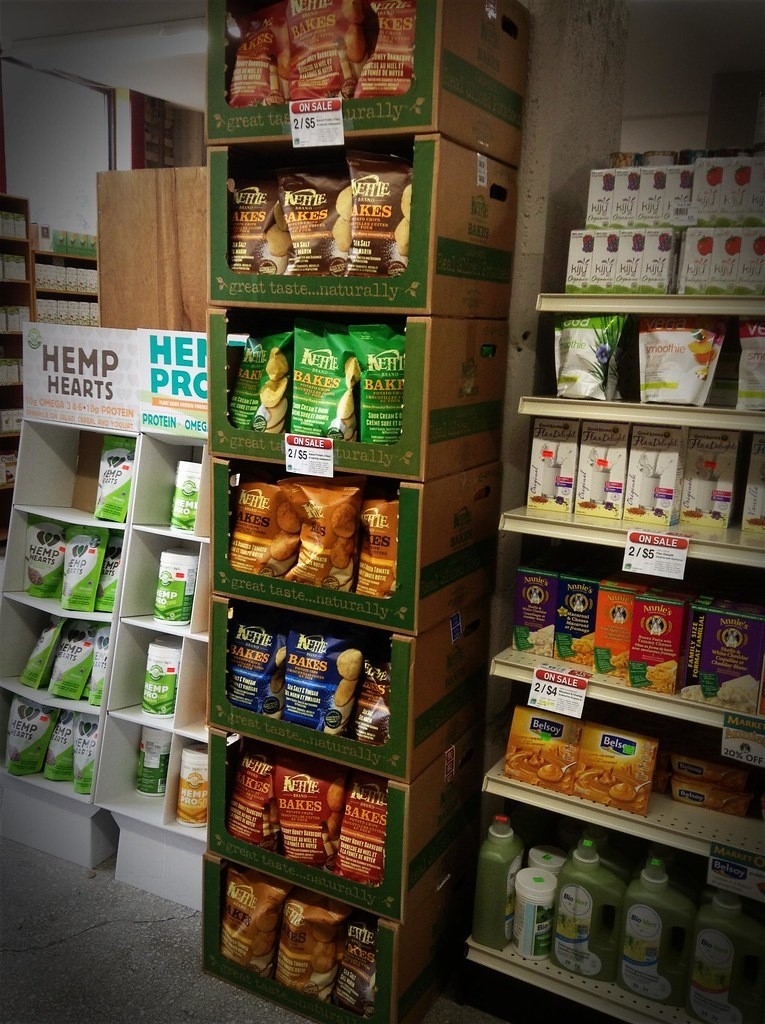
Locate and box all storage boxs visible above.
[502,408,763,824]
[197,0,533,1022]
[563,158,765,300]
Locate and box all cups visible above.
[638,476,660,512]
[540,465,561,499]
[695,478,718,515]
[590,468,610,504]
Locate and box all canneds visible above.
[134,461,210,828]
[512,843,568,960]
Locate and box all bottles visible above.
[550,836,625,982]
[616,858,698,1007]
[473,816,525,948]
[683,890,765,1024]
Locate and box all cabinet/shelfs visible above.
[0,415,209,914]
[459,295,765,1023]
[0,195,105,560]
